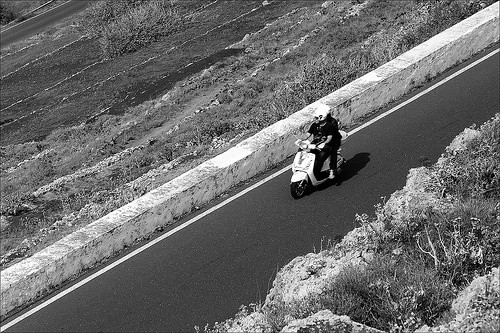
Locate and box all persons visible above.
[318,103,342,179]
[294,107,337,180]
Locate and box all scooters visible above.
[290,130,347,199]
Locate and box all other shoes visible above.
[329,169,337,179]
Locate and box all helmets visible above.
[318,104,331,115]
[313,108,327,122]
